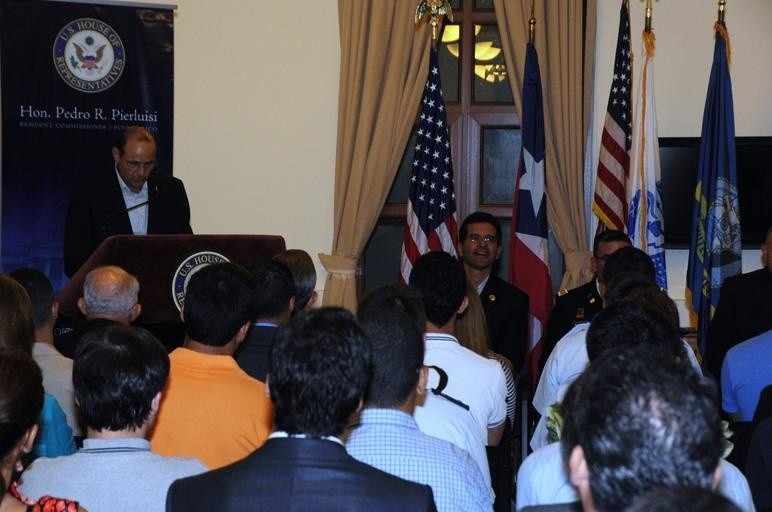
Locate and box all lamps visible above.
[444,22,509,82]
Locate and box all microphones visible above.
[153,184,160,199]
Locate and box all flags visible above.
[592,4,633,236]
[508,42,553,395]
[625,31,667,295]
[686,21,743,363]
[398,48,460,287]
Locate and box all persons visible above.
[63,126,193,279]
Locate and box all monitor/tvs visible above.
[659,136,772,249]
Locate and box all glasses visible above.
[120,155,154,169]
[463,235,497,243]
